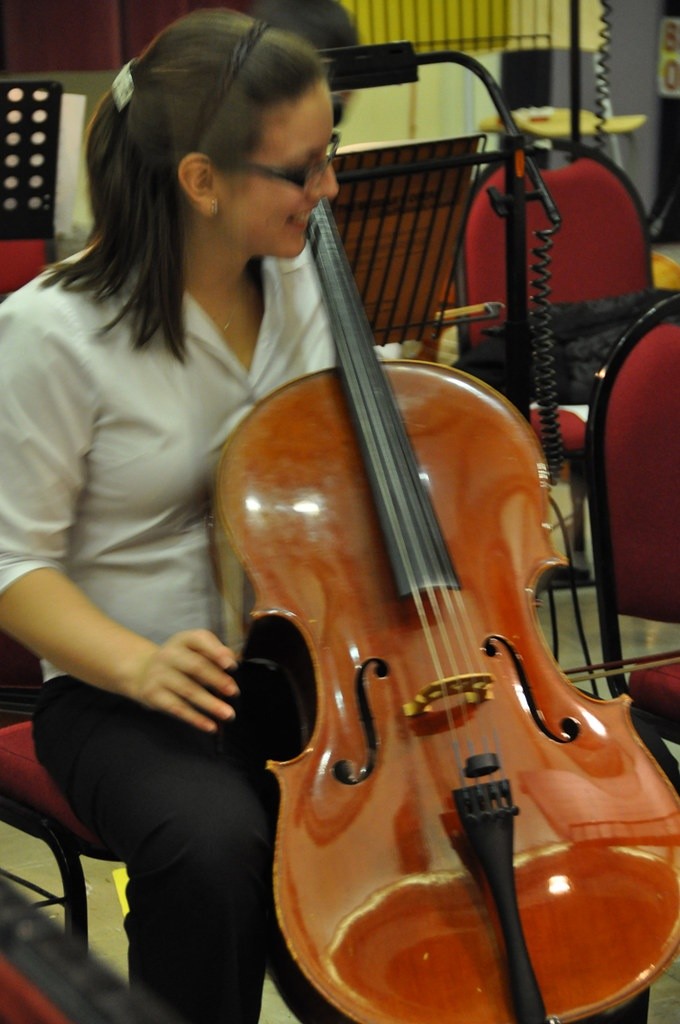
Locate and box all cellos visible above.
[215,197,680,1024]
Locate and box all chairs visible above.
[452,131,660,496]
[583,291,680,789]
[0,715,127,956]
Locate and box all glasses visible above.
[230,130,341,194]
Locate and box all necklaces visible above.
[209,283,253,333]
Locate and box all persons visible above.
[0,6,373,1024]
